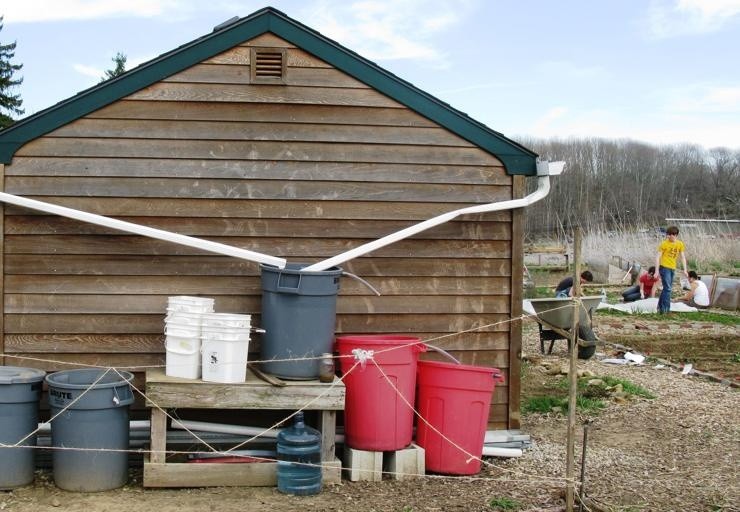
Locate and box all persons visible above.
[653,226,688,314]
[555,271,593,296]
[672,270,709,309]
[622,266,659,301]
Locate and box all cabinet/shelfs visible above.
[523,240,570,272]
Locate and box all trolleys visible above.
[530,296,601,359]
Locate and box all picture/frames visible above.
[710,276,740,310]
[696,272,716,296]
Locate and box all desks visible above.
[141,367,346,488]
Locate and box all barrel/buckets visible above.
[418,360,505,476]
[261,262,341,380]
[337,335,426,450]
[200,311,252,383]
[44,368,134,493]
[276,411,322,496]
[164,296,215,380]
[0,366,46,490]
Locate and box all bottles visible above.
[319,353,335,383]
